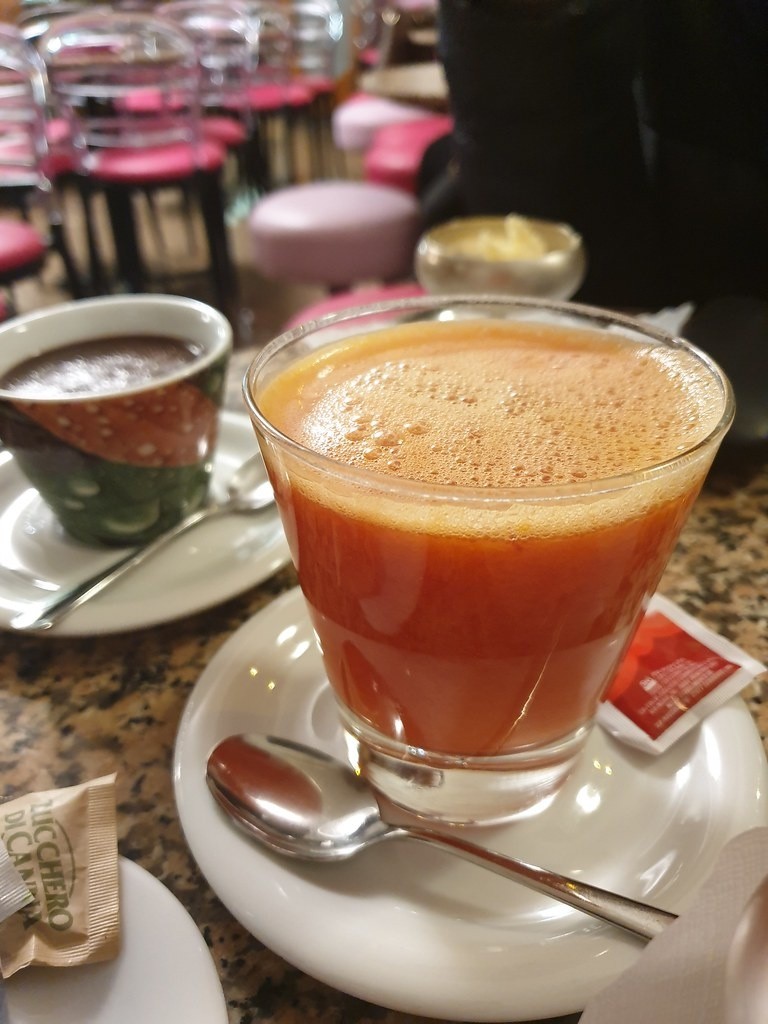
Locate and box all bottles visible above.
[0,1,584,347]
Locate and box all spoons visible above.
[7,450,276,634]
[203,730,680,945]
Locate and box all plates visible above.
[0,407,292,639]
[0,855,230,1024]
[172,583,768,1024]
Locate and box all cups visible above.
[0,291,234,553]
[239,296,739,829]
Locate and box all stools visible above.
[241,26,455,341]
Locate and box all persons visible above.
[418,0,768,312]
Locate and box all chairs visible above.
[0,0,405,324]
